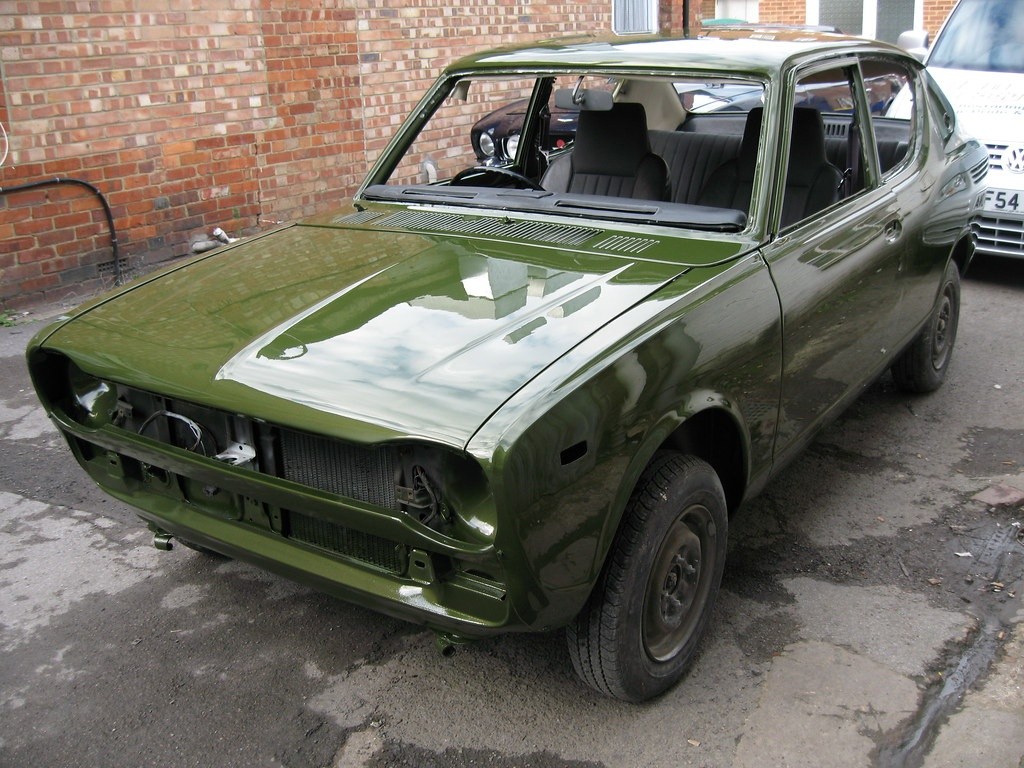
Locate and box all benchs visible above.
[647,127,907,208]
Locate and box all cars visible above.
[885,0,1024,258]
[921,138,988,246]
[30,28,988,704]
[469,24,906,167]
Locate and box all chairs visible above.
[538,103,669,197]
[698,107,842,228]
